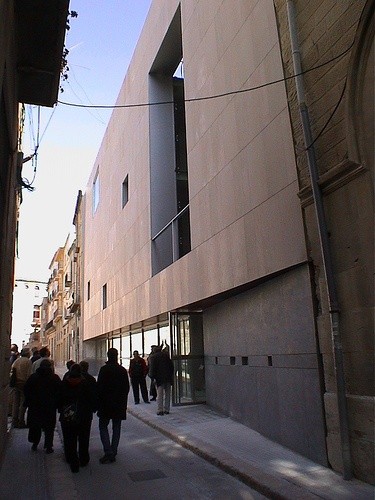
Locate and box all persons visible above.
[8,344,174,473]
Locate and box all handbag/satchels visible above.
[9,367,17,388]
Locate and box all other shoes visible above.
[99,455,117,464]
[46,449,53,454]
[14,424,29,429]
[156,410,169,415]
[31,444,37,450]
